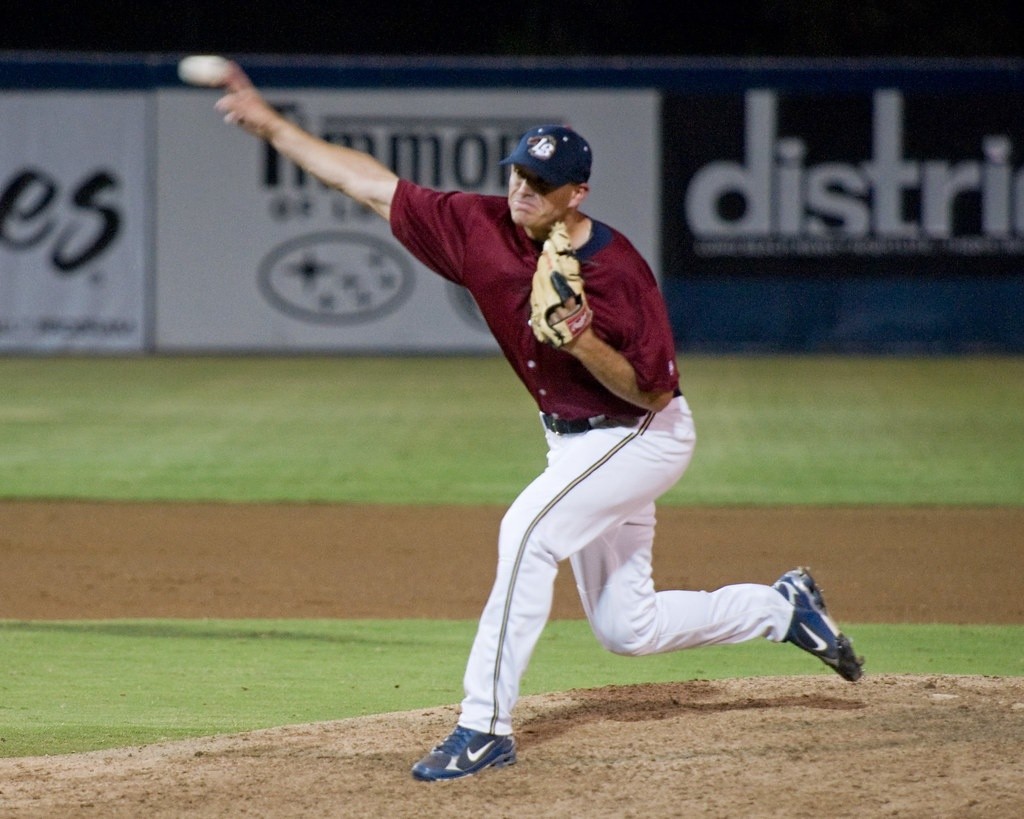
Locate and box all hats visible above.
[498,125,592,185]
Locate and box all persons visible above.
[213,59,863,779]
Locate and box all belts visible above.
[541,413,607,434]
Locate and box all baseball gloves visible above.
[528,218,593,351]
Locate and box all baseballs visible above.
[177,53,232,89]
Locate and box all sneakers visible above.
[411,725,517,781]
[771,565,865,681]
